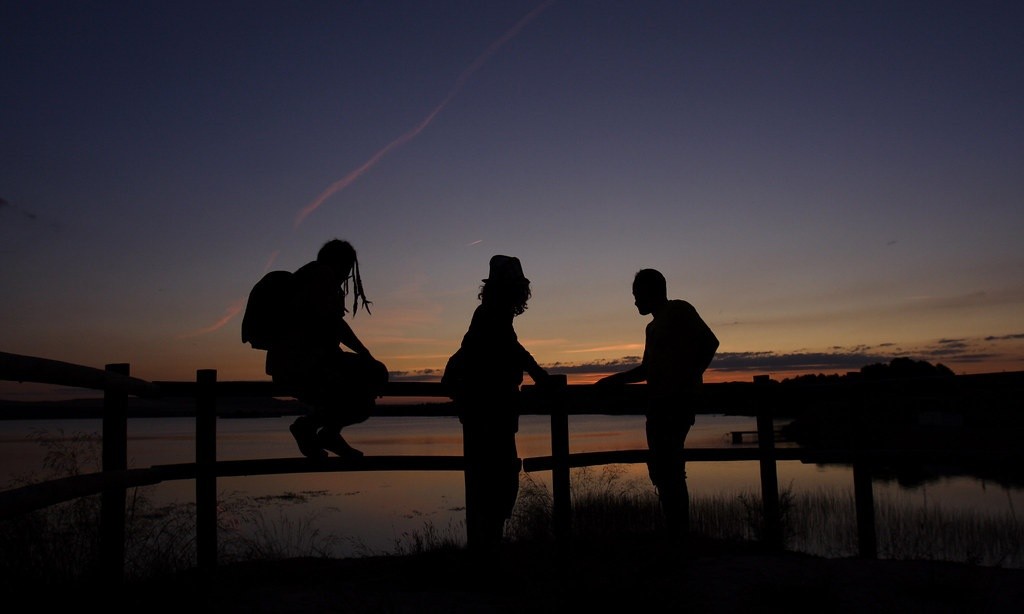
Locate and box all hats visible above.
[482,255,530,287]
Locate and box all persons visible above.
[460,254,565,544]
[591,268,719,549]
[263,240,387,461]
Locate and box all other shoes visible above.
[289,416,328,457]
[316,427,363,458]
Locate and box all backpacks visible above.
[242,270,300,349]
